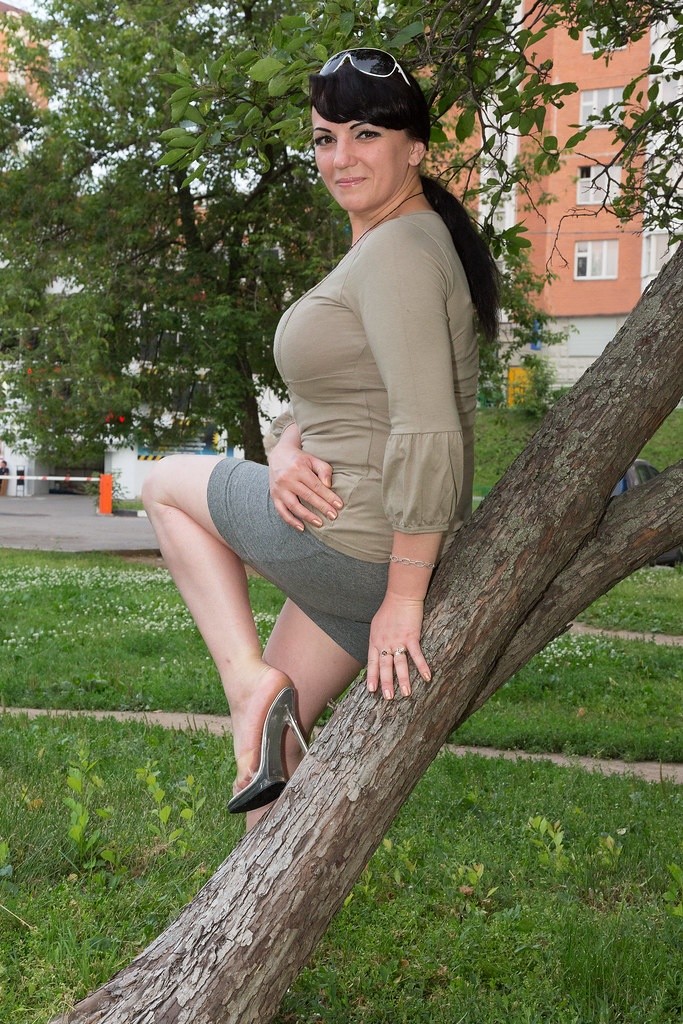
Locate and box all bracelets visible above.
[388,554,435,569]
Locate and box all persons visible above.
[140,46,505,833]
[0,460,9,491]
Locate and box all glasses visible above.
[319,47,413,87]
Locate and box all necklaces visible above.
[332,191,423,271]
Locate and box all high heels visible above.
[227,684,314,814]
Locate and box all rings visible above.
[380,649,394,657]
[393,647,407,655]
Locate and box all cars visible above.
[609,460,683,568]
